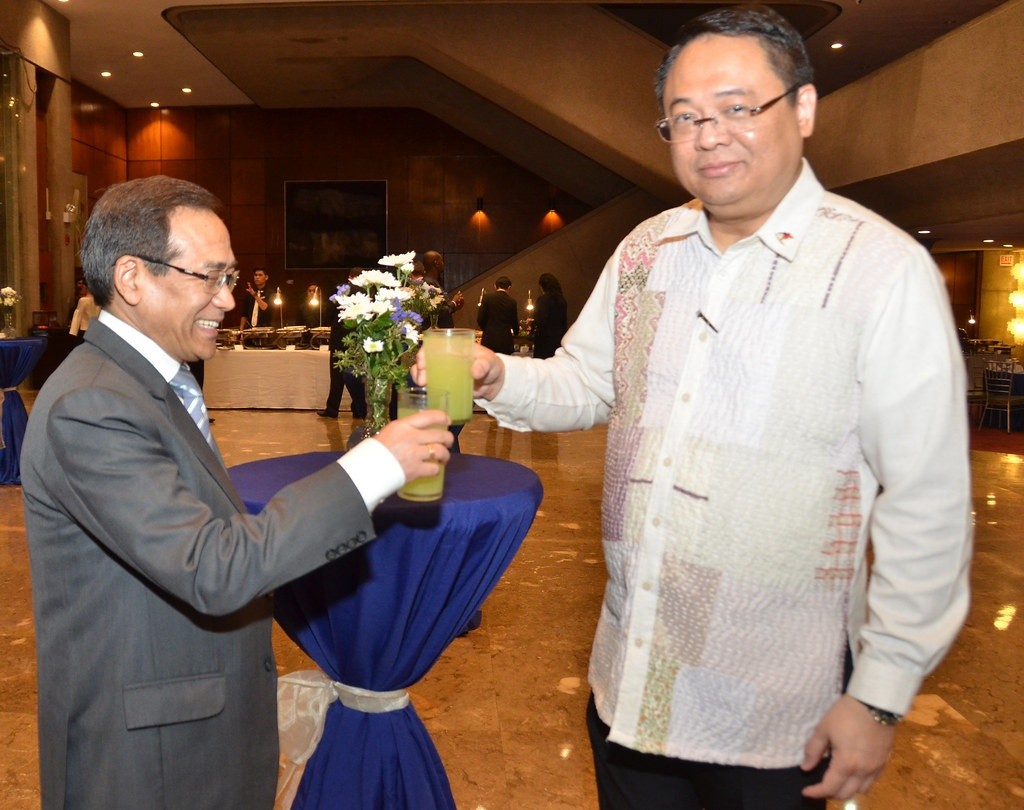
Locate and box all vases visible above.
[347,379,393,450]
[1,313,17,338]
[426,314,438,330]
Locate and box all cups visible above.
[424,328,476,424]
[396,387,450,501]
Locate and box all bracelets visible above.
[868,706,897,726]
[451,301,457,307]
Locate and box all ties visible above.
[250,287,261,326]
[167,365,221,464]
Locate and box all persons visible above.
[19,175,454,810]
[68,278,101,336]
[316,267,368,419]
[530,273,567,359]
[409,251,465,331]
[477,276,519,355]
[240,269,274,326]
[409,3,975,810]
[295,284,328,327]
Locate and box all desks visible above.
[0,335,48,485]
[202,348,353,410]
[225,451,543,810]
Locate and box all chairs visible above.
[976,361,1024,433]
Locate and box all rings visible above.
[427,444,435,460]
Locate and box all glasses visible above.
[113,253,242,294]
[657,80,804,143]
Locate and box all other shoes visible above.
[316,409,337,420]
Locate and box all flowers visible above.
[0,286,22,313]
[402,276,448,330]
[327,251,422,435]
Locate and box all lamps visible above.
[526,290,535,310]
[478,288,484,306]
[967,310,976,324]
[309,287,322,327]
[274,286,283,328]
